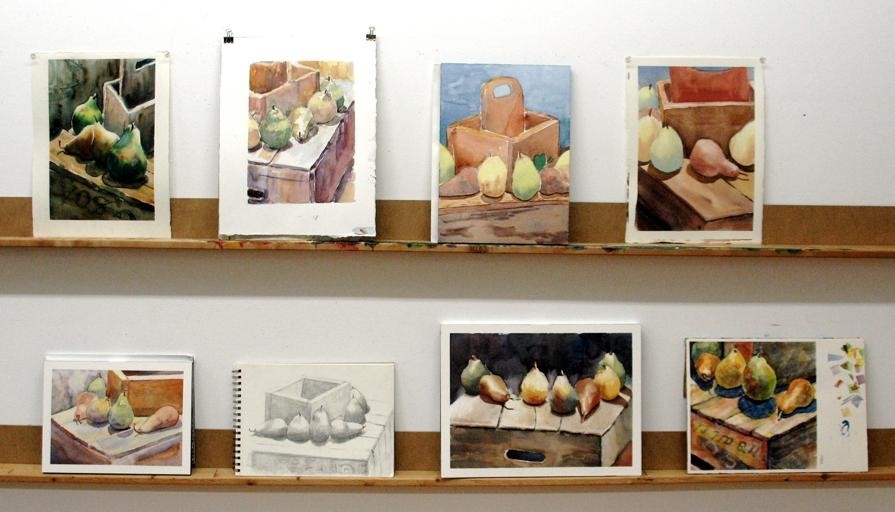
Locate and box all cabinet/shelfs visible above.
[1,196,895,492]
